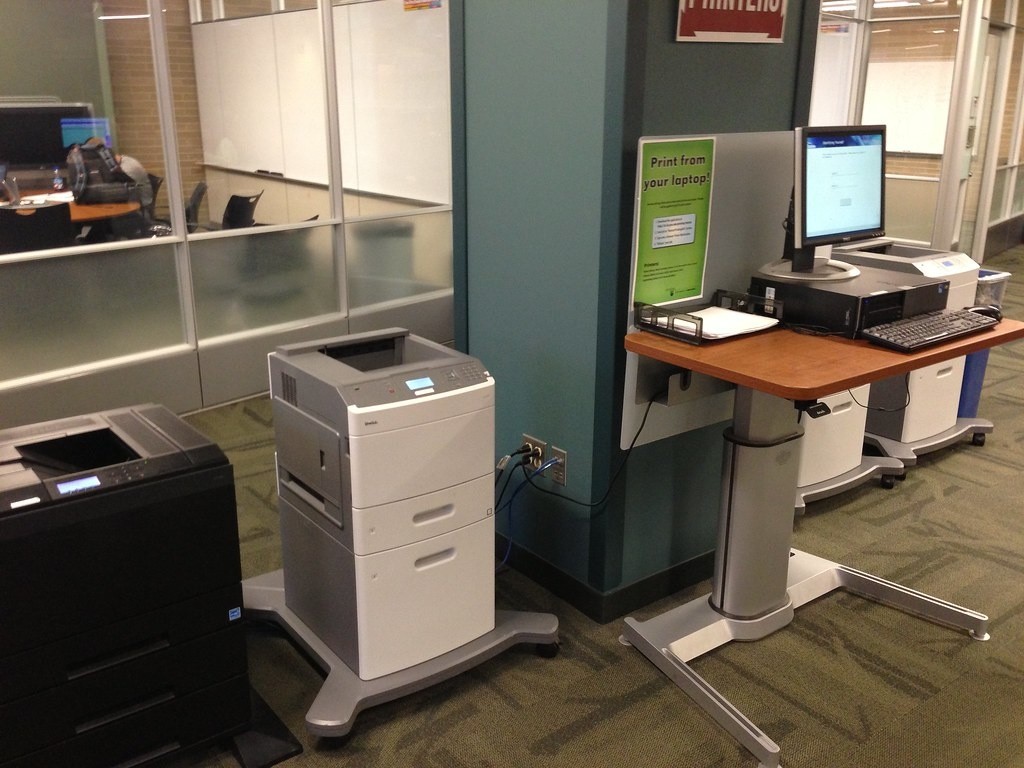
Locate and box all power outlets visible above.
[522,433,547,477]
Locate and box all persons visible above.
[82,137,155,241]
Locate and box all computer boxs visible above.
[751,264,952,341]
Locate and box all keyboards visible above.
[863,309,1000,352]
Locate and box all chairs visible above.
[201,190,414,305]
[105,173,208,238]
[0,201,72,253]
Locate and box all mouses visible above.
[963,305,1003,322]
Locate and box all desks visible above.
[0,189,139,223]
[623,318,1024,768]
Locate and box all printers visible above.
[269,327,497,679]
[0,401,258,768]
[830,239,980,444]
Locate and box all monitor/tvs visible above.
[61,118,113,149]
[768,125,886,281]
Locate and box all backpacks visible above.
[66,137,135,203]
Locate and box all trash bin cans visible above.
[957,268,1012,417]
